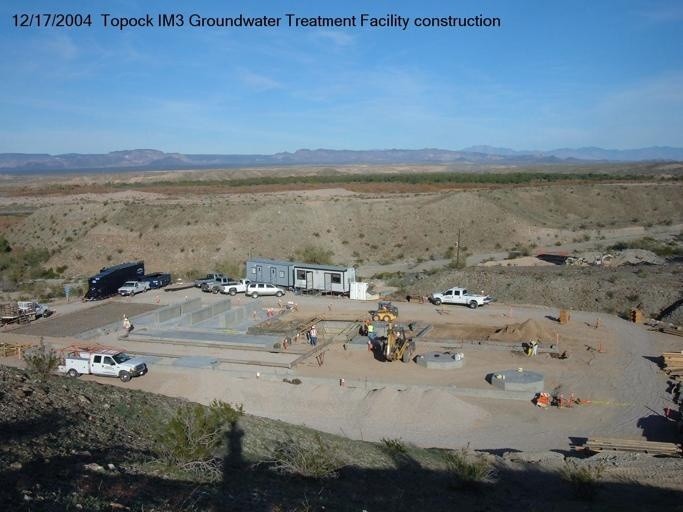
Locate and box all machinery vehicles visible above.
[369,300,398,321]
[367,321,414,363]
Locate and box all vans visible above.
[245,282,284,298]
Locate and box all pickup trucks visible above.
[218,279,251,295]
[200,277,234,293]
[58,349,146,382]
[194,273,225,287]
[17,301,48,318]
[118,280,151,296]
[428,288,491,308]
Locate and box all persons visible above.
[310,325,317,346]
[123,319,131,331]
